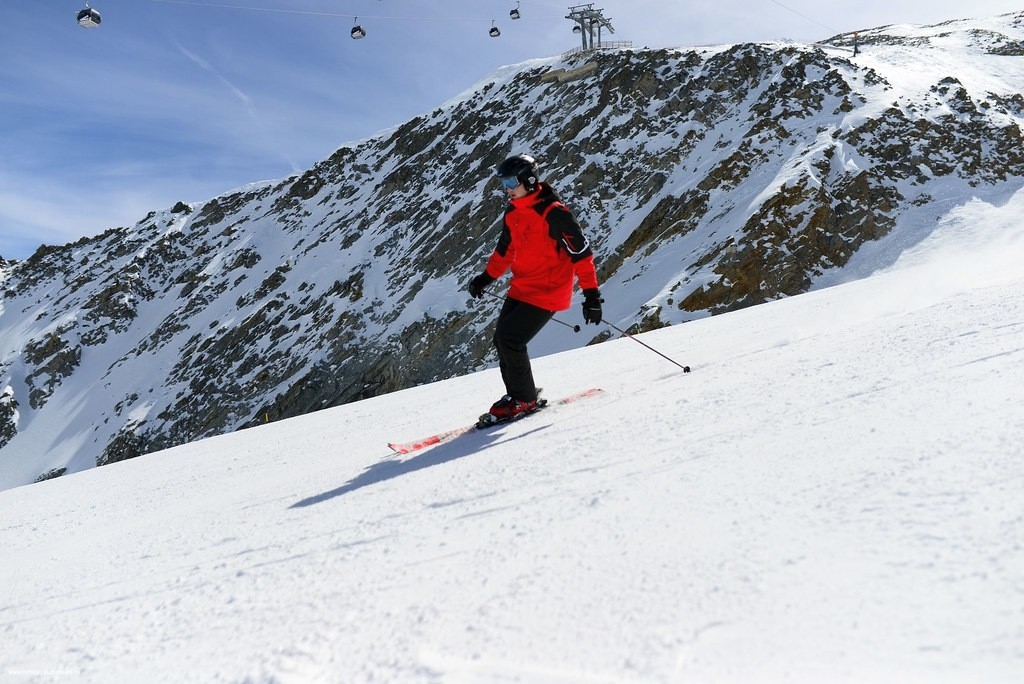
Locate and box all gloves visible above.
[581,291,605,325]
[469,269,497,299]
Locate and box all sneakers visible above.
[489,394,536,418]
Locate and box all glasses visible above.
[500,165,529,192]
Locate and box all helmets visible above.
[497,153,540,193]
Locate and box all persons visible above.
[469,155,605,419]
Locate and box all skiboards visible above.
[386,389,604,455]
